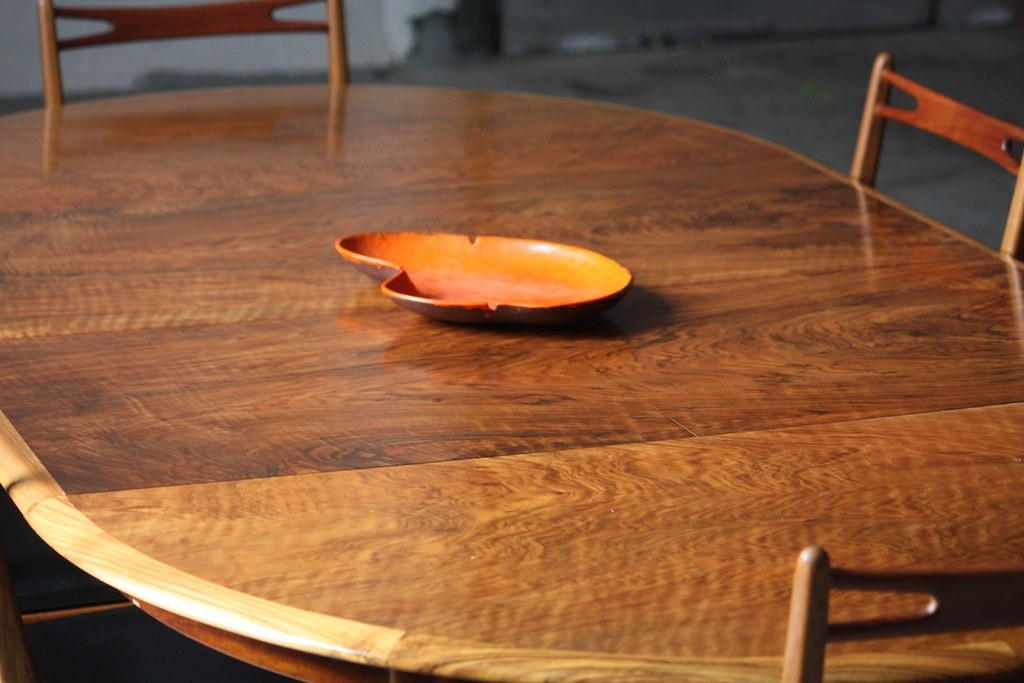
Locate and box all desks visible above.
[0,85,1023,683]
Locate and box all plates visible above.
[334,231,634,325]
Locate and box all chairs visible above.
[35,0,347,106]
[847,53,1023,256]
[781,546,1023,683]
